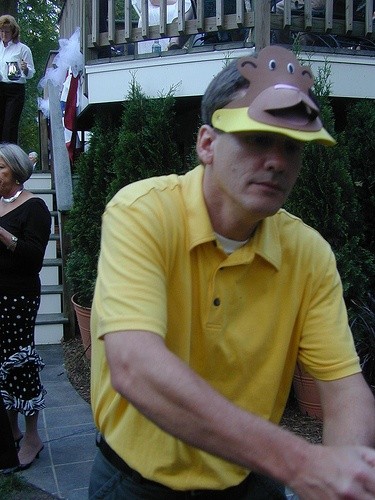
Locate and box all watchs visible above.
[6,235,18,250]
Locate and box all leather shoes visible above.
[0,465,21,476]
[94,432,255,500]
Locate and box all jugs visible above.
[4,62,24,81]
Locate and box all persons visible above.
[75,132,83,153]
[28,151,40,172]
[0,144,52,475]
[88,45,375,500]
[137,0,327,53]
[0,15,36,145]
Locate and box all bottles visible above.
[152,39,161,57]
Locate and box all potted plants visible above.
[66,246,101,362]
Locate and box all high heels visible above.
[13,435,45,473]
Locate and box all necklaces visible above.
[1,183,25,203]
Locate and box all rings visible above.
[23,66,24,69]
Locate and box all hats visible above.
[211,42,336,145]
